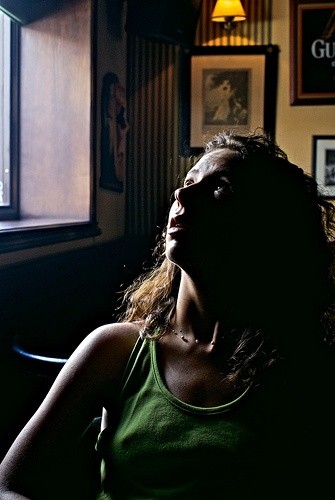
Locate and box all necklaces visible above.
[162,323,241,348]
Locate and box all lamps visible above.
[209,0,247,46]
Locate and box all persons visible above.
[1,128,334,500]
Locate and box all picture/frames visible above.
[289,0,335,107]
[311,134,335,201]
[179,44,279,157]
